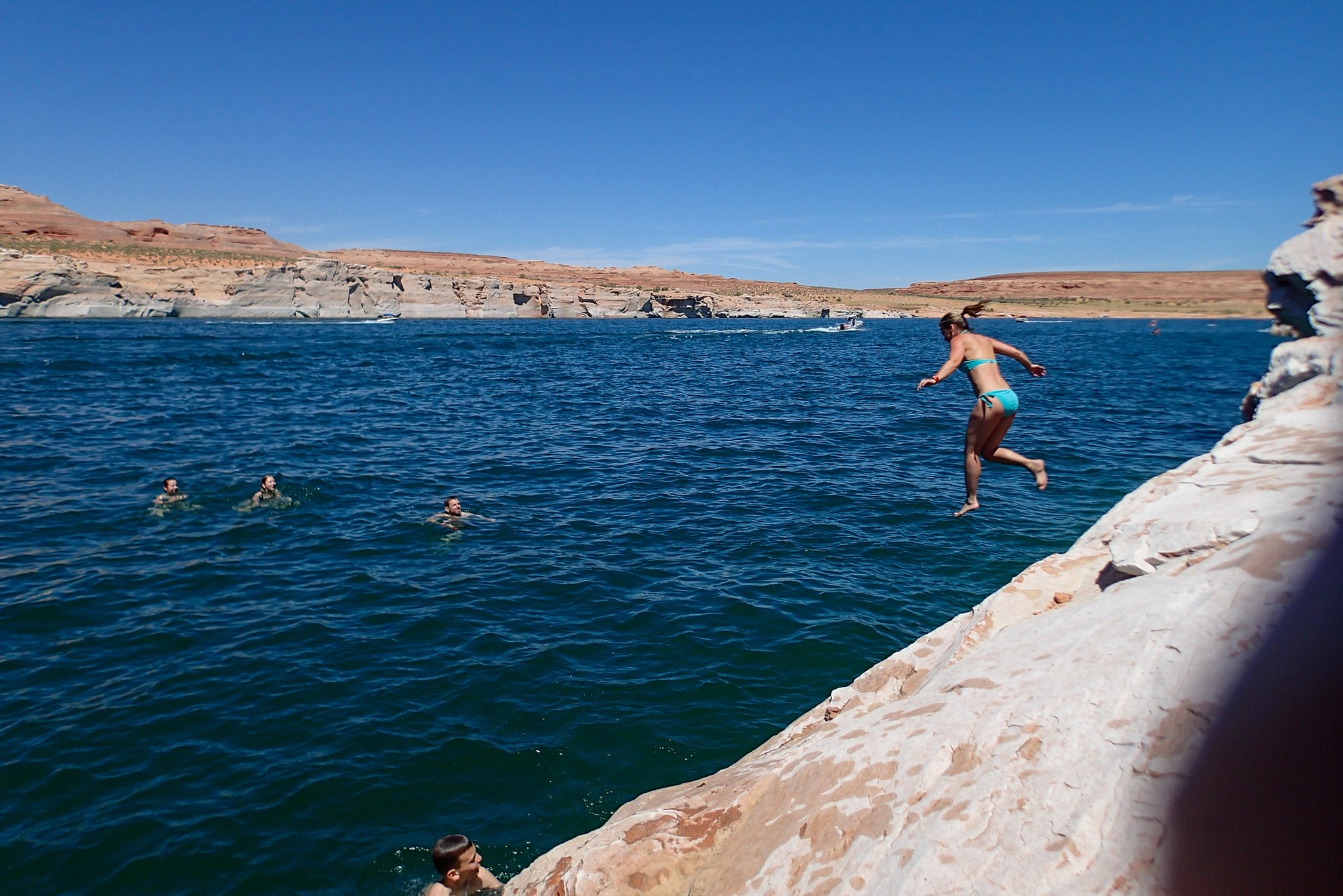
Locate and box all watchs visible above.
[932,375,939,384]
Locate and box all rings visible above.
[921,380,924,383]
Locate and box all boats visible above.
[375,313,399,322]
[834,316,864,331]
[1015,317,1028,323]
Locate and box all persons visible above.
[156,477,188,501]
[425,496,496,521]
[917,299,1048,518]
[252,475,283,501]
[426,835,506,896]
